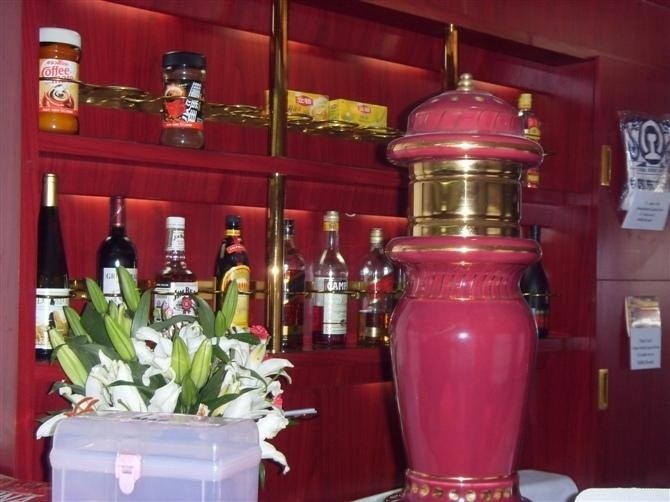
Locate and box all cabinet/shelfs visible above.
[19,0,575,378]
[532,53,669,488]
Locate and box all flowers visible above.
[30,268,305,475]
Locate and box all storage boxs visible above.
[43,409,263,502]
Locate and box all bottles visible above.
[213,214,252,338]
[153,216,200,330]
[522,223,551,339]
[282,218,306,352]
[310,210,350,351]
[357,226,397,347]
[517,92,545,191]
[33,171,72,362]
[95,194,139,310]
